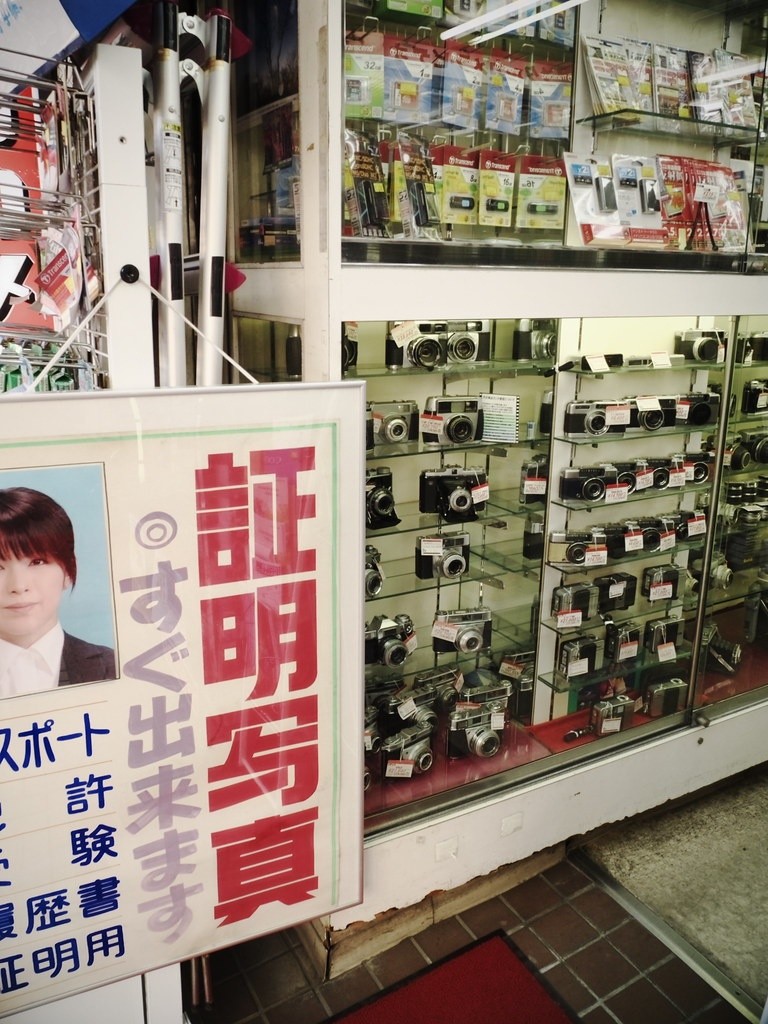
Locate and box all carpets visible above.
[319,928,588,1024]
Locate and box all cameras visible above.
[285,320,768,790]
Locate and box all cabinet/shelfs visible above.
[225,0,768,832]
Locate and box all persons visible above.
[1,486,121,691]
